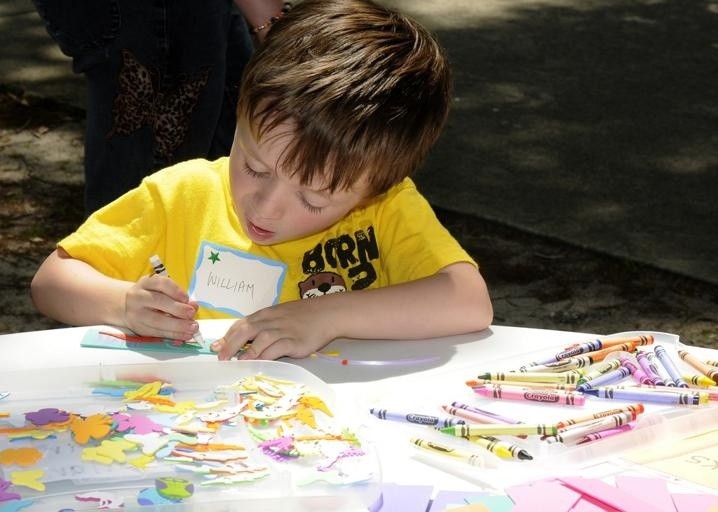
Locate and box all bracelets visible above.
[250,2,292,34]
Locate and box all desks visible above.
[0,317,718,511]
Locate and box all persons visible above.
[28,1,293,221]
[28,0,496,362]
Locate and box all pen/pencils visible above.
[149,254,208,349]
[370,335,718,466]
